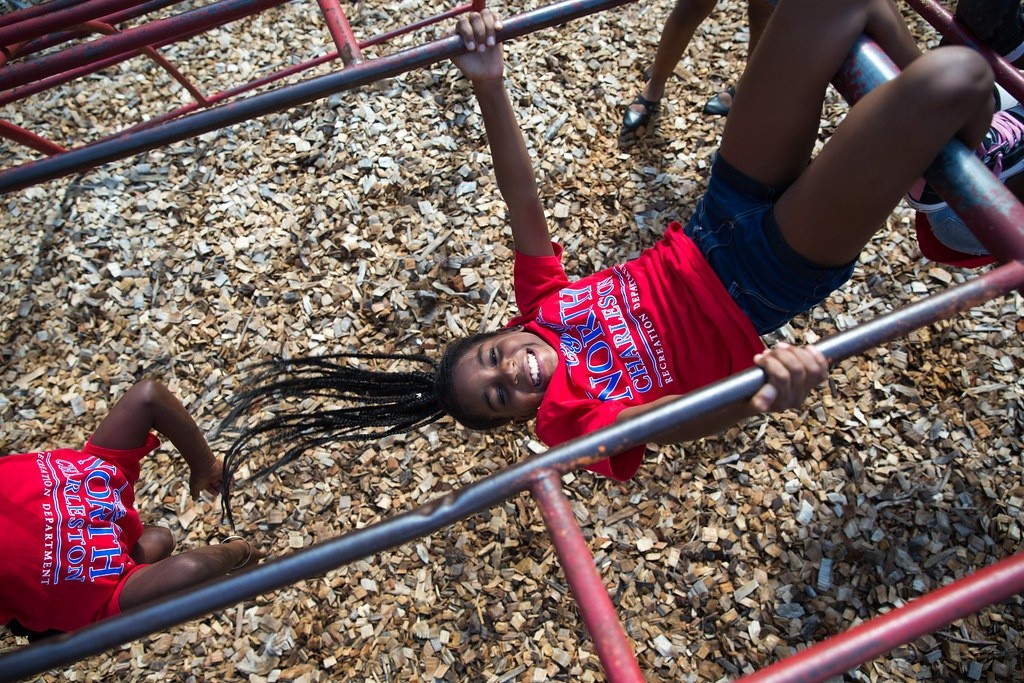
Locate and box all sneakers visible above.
[904,103,1024,213]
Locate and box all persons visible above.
[0,380,269,636]
[620,0,780,138]
[915,0,1024,266]
[213,0,1024,486]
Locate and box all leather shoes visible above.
[623,88,663,128]
[704,86,736,115]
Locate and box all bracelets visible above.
[221,536,253,569]
